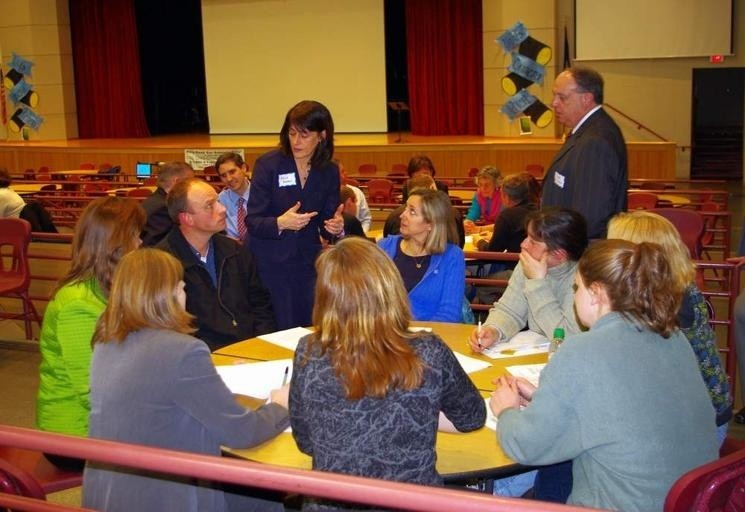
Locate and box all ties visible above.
[235,196,248,243]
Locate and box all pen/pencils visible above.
[283,366,288,385]
[478,313,481,348]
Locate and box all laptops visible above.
[136,163,152,178]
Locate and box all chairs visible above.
[662,448,744,511]
[204,165,226,193]
[341,165,732,327]
[2,458,82,500]
[1,218,42,340]
[2,163,157,231]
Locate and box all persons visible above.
[732,287,745,426]
[486,237,721,510]
[0,166,25,219]
[37,194,145,471]
[287,235,484,512]
[144,175,279,348]
[536,62,630,243]
[371,189,469,322]
[79,246,293,510]
[242,99,349,330]
[469,209,735,497]
[467,209,591,354]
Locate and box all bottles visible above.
[547,326,568,364]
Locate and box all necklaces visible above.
[294,159,310,183]
[405,240,431,272]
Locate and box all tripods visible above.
[395,109,408,142]
